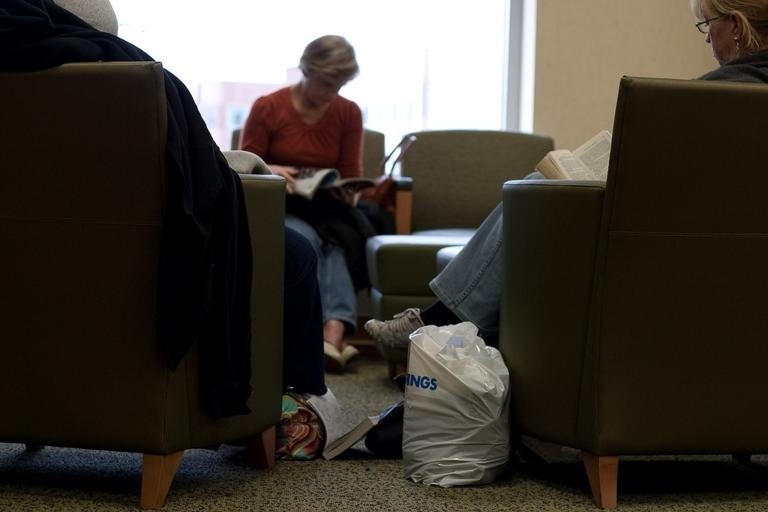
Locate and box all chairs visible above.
[229,128,414,344]
[497,75,768,509]
[0,58,287,510]
[399,130,555,238]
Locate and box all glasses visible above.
[695,14,722,33]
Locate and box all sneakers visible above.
[364,307,425,350]
[324,340,358,373]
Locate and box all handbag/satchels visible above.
[276,390,323,461]
[365,406,403,460]
[357,177,396,221]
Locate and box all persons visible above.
[365,0,768,354]
[55,0,327,398]
[238,34,362,374]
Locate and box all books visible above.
[305,385,380,463]
[296,165,374,201]
[535,128,613,181]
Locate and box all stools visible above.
[364,235,484,378]
